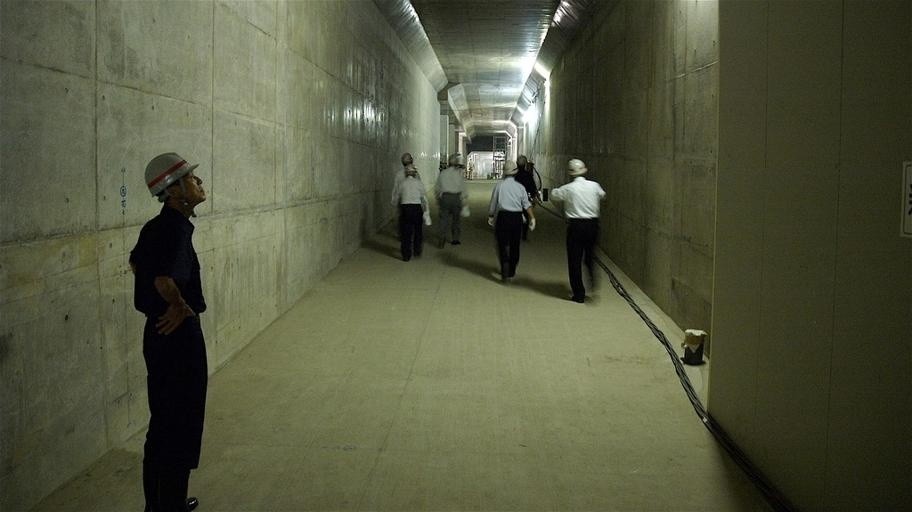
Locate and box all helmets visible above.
[401,152,414,166]
[567,158,588,176]
[144,152,199,197]
[517,156,527,166]
[449,155,458,167]
[405,164,417,177]
[503,160,519,176]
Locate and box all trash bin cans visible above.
[684,329,706,365]
[543,188,548,201]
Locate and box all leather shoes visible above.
[452,240,460,244]
[185,497,199,512]
[572,296,584,303]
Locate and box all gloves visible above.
[528,218,536,232]
[488,217,495,228]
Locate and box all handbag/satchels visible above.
[421,210,432,227]
[461,206,470,217]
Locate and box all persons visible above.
[487,160,537,284]
[390,162,427,262]
[551,158,608,304]
[433,153,469,249]
[469,158,475,180]
[126,150,210,512]
[515,152,540,243]
[390,149,421,242]
